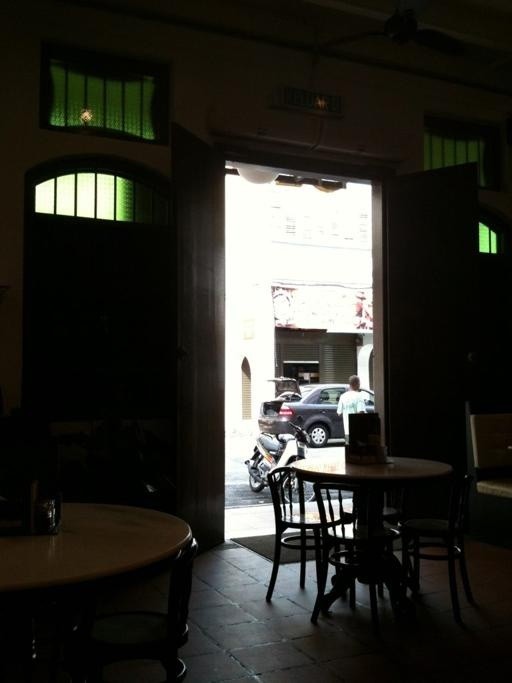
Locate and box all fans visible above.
[311,0,466,57]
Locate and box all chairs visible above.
[464,399,511,551]
[69,535,198,683]
[265,455,479,628]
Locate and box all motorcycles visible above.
[244,414,318,503]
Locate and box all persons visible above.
[335,373,367,445]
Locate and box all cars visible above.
[258,375,373,448]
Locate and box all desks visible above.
[0,501,193,683]
